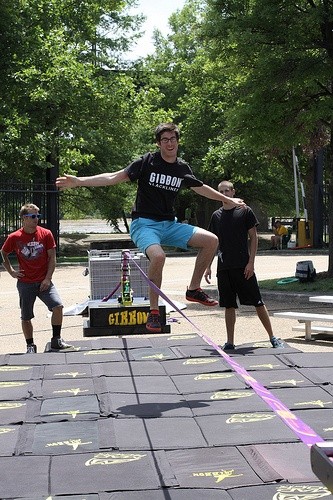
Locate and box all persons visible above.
[55,124,245,331]
[208,181,282,349]
[0,203,74,354]
[271,221,287,250]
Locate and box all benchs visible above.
[273,311,333,341]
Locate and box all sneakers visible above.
[270,337,284,349]
[26,345,37,354]
[186,286,218,306]
[146,309,161,332]
[214,344,235,354]
[50,339,74,352]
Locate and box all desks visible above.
[309,295,333,303]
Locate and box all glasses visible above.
[160,137,177,143]
[22,214,42,219]
